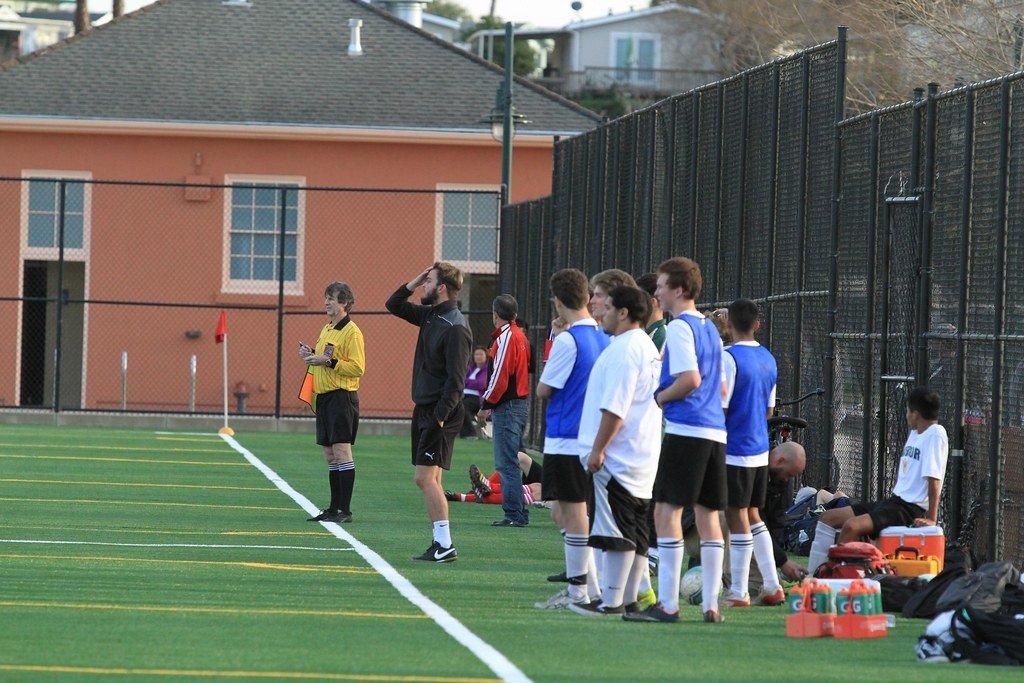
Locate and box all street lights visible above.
[476,83,538,295]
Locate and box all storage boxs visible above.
[832,610,889,640]
[786,613,836,636]
[877,523,945,573]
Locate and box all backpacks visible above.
[813,542,1024,667]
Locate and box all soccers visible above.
[681,566,724,605]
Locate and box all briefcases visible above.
[879,525,945,576]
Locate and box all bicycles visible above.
[768,385,827,507]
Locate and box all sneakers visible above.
[546,572,568,582]
[623,602,681,623]
[492,519,529,527]
[413,539,458,563]
[308,510,352,522]
[469,464,492,503]
[536,590,657,616]
[752,586,785,605]
[717,590,752,608]
[702,609,724,623]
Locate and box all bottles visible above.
[788,577,882,615]
[885,614,896,627]
[780,569,807,579]
[798,530,809,545]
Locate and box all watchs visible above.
[325,358,333,368]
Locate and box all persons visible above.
[298,282,365,523]
[385,261,474,563]
[921,324,983,513]
[458,257,811,624]
[808,387,948,577]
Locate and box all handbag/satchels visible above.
[781,495,849,557]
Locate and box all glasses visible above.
[550,294,555,302]
[713,310,721,318]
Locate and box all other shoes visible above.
[463,490,474,494]
[444,489,456,501]
